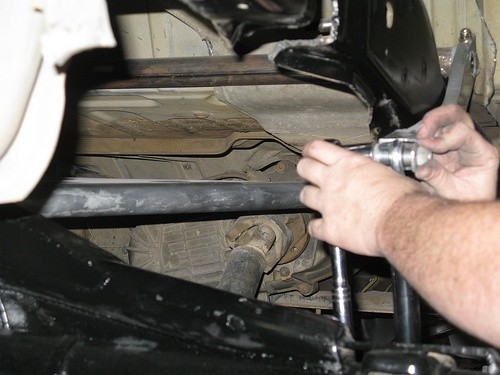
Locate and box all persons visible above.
[297,104,499,350]
